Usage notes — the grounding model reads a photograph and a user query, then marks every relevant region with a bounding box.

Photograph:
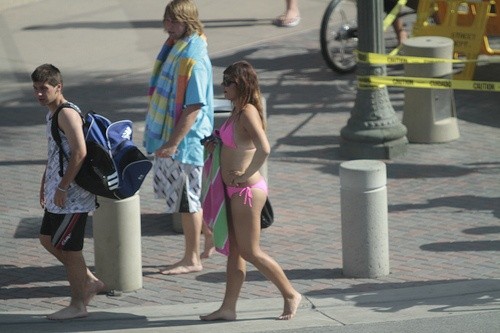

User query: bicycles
[319,0,359,75]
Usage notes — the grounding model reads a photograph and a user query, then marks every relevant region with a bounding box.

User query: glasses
[221,80,235,86]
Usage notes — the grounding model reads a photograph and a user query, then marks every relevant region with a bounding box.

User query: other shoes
[278,14,300,26]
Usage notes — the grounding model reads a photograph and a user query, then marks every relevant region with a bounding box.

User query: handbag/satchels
[261,199,273,228]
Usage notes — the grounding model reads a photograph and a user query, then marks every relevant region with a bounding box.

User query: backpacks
[49,102,152,209]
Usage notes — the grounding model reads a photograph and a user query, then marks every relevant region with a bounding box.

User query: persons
[30,63,105,322]
[197,59,304,321]
[271,0,302,27]
[380,1,422,45]
[142,0,218,275]
